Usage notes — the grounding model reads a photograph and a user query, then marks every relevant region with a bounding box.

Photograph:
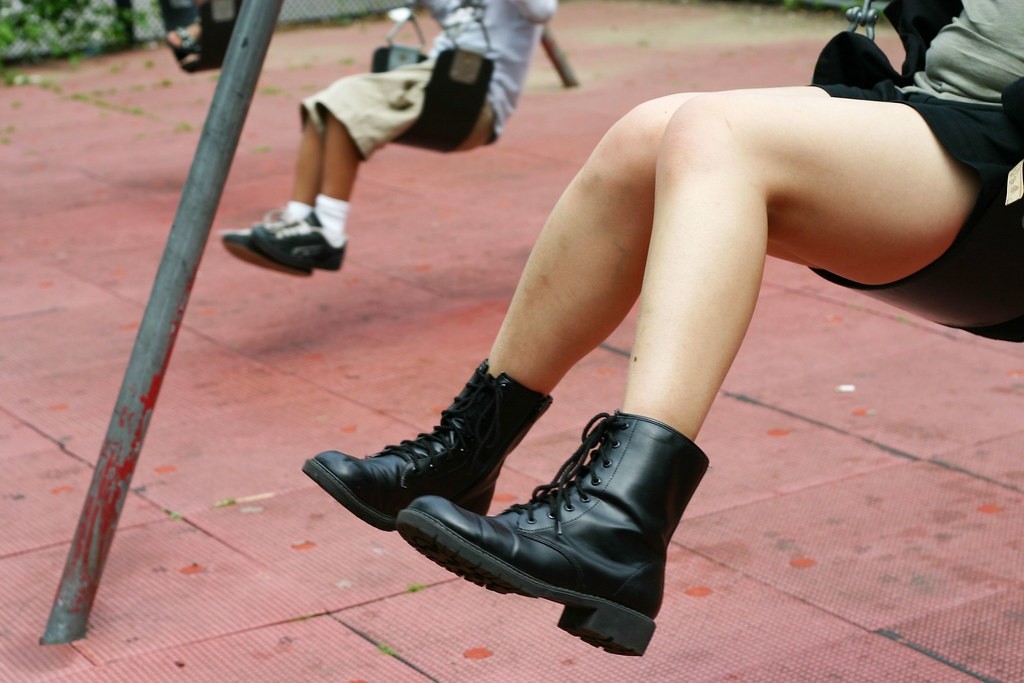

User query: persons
[302,0,1024,658]
[221,0,559,277]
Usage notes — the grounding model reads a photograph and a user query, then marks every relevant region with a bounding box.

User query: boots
[396,408,710,657]
[301,358,553,534]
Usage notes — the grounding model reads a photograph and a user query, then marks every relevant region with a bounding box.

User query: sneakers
[251,210,347,271]
[221,210,313,276]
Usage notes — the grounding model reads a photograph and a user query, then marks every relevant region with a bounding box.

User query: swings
[804,0,1024,330]
[371,1,498,155]
